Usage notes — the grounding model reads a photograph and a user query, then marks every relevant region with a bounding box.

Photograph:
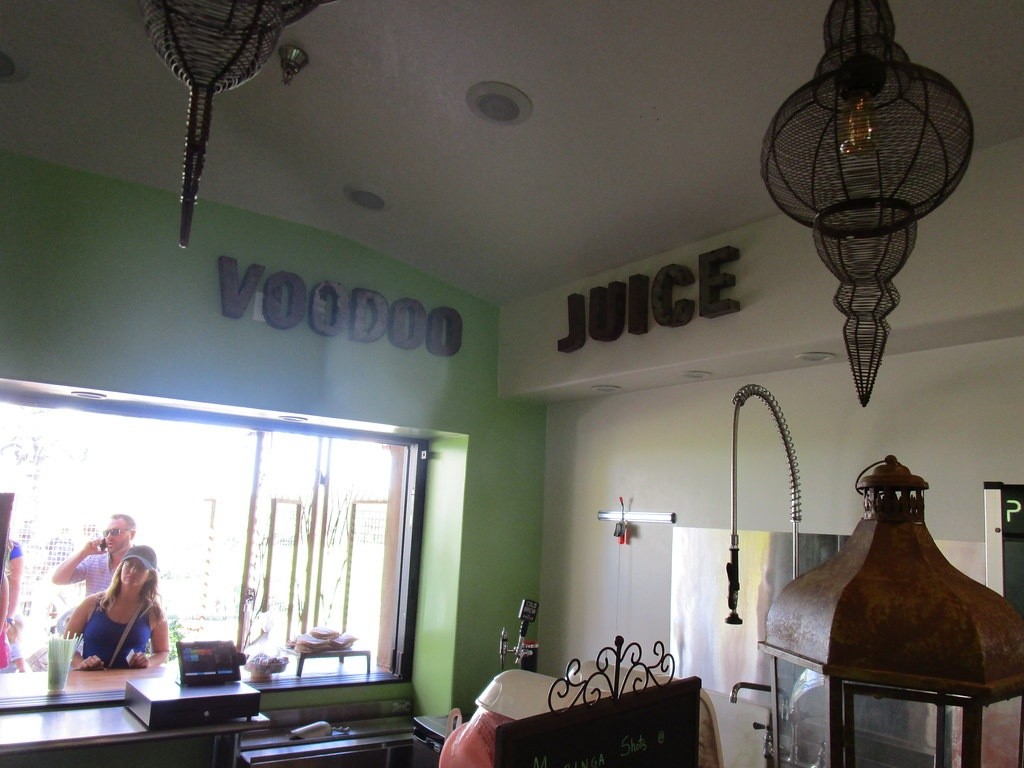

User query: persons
[0,578,10,674]
[5,540,23,631]
[0,615,25,673]
[51,513,135,596]
[62,542,170,671]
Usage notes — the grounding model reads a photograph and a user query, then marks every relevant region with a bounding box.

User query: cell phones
[100,539,107,552]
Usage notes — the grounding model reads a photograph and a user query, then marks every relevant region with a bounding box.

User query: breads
[287,627,357,654]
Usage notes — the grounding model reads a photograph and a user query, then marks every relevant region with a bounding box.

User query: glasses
[103,528,130,536]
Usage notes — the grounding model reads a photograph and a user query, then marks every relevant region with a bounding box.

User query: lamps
[598,510,676,524]
[758,0,975,408]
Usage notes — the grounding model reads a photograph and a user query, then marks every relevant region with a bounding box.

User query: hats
[121,545,158,569]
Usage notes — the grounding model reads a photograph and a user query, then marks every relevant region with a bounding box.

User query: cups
[47,654,72,695]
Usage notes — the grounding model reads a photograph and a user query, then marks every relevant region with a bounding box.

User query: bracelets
[6,618,15,625]
